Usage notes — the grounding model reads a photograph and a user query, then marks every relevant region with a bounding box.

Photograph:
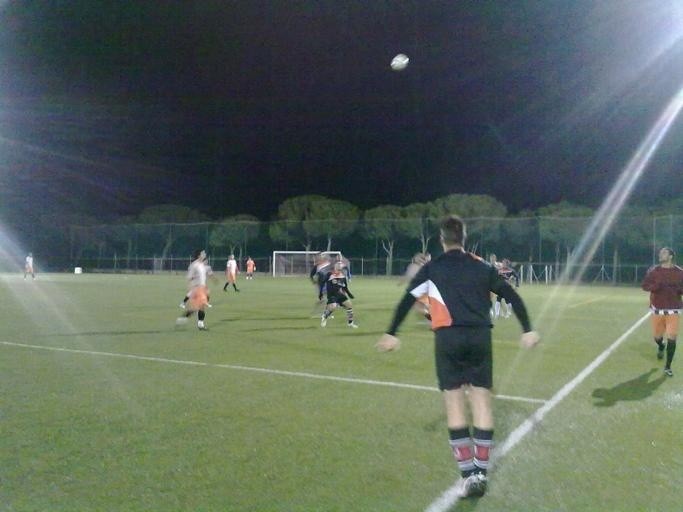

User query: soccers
[390,53,410,71]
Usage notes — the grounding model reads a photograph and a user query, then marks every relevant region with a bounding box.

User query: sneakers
[488,311,512,320]
[175,276,252,332]
[656,342,665,359]
[23,278,37,281]
[459,472,488,499]
[347,322,359,329]
[320,310,335,328]
[663,367,674,378]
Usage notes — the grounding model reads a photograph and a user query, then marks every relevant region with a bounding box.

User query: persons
[175,247,213,332]
[372,213,539,499]
[22,252,36,281]
[309,253,335,304]
[319,260,360,330]
[640,249,682,377]
[222,254,241,293]
[404,249,519,324]
[202,258,214,305]
[333,252,351,284]
[245,254,256,281]
